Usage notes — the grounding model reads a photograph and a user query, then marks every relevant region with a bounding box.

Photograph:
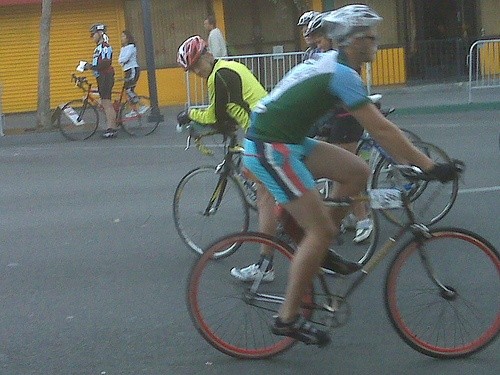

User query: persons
[241,4,456,345]
[77,23,118,138]
[118,30,151,118]
[296,11,375,242]
[203,15,229,61]
[176,34,277,282]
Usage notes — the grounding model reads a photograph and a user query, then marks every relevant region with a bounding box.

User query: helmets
[304,11,333,38]
[297,9,320,26]
[176,35,209,72]
[89,23,107,32]
[321,4,384,49]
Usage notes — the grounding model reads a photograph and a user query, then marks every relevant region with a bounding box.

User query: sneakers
[352,217,375,243]
[318,248,364,277]
[271,313,331,348]
[230,262,275,283]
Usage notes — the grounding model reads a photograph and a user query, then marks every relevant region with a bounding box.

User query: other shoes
[102,128,118,138]
[137,105,149,114]
[125,109,137,118]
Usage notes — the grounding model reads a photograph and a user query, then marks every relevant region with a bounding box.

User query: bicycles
[57,73,159,139]
[173,115,378,268]
[349,92,432,204]
[187,163,500,357]
[354,107,460,226]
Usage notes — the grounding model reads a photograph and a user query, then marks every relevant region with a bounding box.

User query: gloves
[177,110,191,126]
[431,162,462,183]
